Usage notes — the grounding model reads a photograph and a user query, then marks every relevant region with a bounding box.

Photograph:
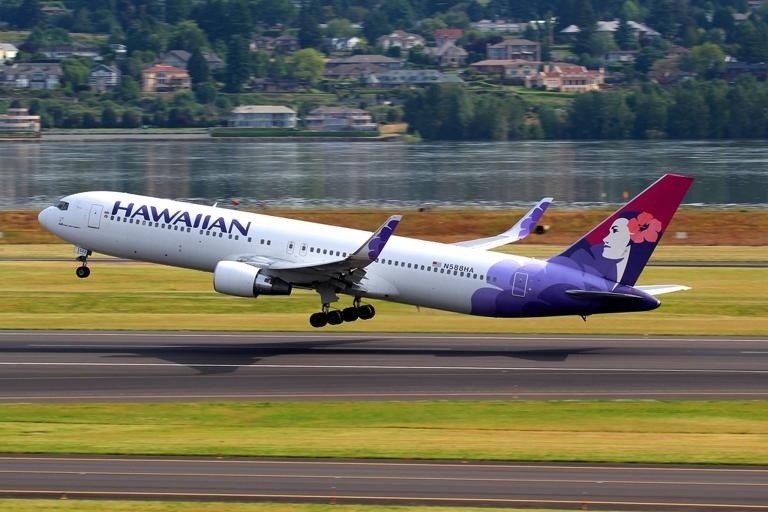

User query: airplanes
[36,172,697,330]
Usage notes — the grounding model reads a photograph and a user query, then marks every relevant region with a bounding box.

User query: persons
[601,210,663,293]
